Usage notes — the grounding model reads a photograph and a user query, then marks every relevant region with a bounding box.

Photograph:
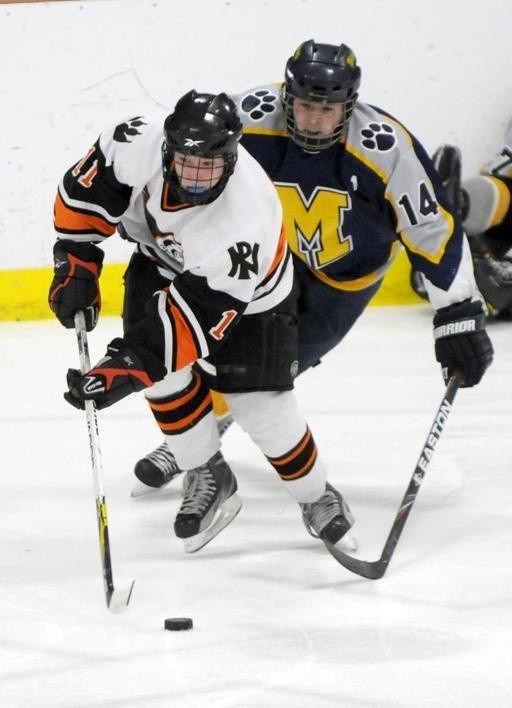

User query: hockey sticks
[322,369,461,579]
[74,310,133,613]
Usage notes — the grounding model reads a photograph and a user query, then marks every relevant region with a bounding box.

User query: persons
[45,87,356,549]
[408,137,512,319]
[132,37,494,489]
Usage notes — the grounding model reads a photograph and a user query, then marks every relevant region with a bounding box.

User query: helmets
[161,88,243,204]
[279,39,361,151]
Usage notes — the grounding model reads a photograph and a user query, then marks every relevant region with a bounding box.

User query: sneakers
[136,442,181,488]
[431,143,466,223]
[298,481,355,545]
[175,449,238,538]
[467,232,511,312]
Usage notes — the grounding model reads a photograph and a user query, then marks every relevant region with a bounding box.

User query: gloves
[49,240,105,332]
[433,300,493,388]
[64,339,167,410]
